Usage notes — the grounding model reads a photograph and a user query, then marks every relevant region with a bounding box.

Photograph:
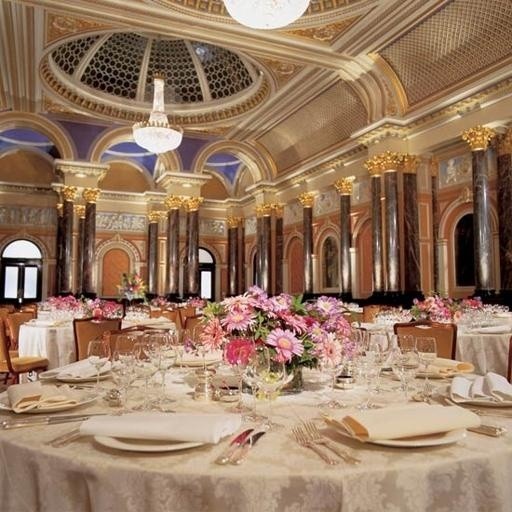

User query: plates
[92,435,208,455]
[321,423,468,448]
[401,357,511,409]
[57,373,110,383]
[1,383,105,411]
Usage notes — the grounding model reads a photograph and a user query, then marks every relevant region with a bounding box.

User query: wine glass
[86,328,437,429]
[36,297,150,325]
[375,294,511,325]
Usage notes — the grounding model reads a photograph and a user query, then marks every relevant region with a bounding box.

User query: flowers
[195,283,359,373]
[114,268,150,297]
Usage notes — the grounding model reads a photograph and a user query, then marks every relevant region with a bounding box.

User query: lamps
[221,0,311,31]
[128,30,186,157]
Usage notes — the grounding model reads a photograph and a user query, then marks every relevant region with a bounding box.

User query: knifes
[232,429,265,465]
[0,411,108,430]
[215,427,255,463]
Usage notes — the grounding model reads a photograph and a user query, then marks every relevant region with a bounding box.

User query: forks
[302,420,359,464]
[289,425,337,464]
